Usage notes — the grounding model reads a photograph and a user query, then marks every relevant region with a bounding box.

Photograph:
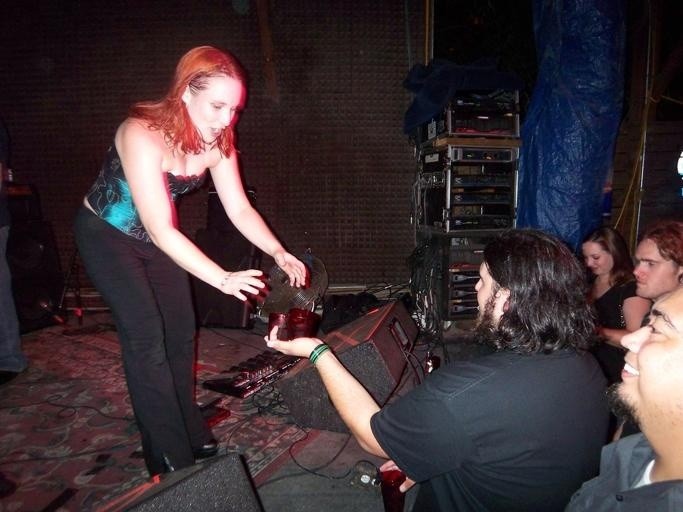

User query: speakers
[276,300,418,434]
[103,450,261,512]
[3,221,58,335]
[195,229,259,329]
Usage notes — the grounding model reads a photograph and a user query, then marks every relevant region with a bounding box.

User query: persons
[564,282,683,512]
[74,45,307,474]
[581,225,653,386]
[0,122,30,389]
[264,229,611,512]
[611,219,683,446]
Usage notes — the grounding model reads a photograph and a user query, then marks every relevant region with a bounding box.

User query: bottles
[423,350,434,377]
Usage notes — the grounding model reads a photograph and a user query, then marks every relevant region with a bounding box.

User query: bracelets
[309,343,331,364]
[218,270,236,293]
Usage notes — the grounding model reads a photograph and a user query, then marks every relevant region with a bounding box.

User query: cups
[377,470,407,511]
[266,309,321,346]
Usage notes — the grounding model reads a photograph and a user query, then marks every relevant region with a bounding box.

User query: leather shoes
[193,439,217,457]
